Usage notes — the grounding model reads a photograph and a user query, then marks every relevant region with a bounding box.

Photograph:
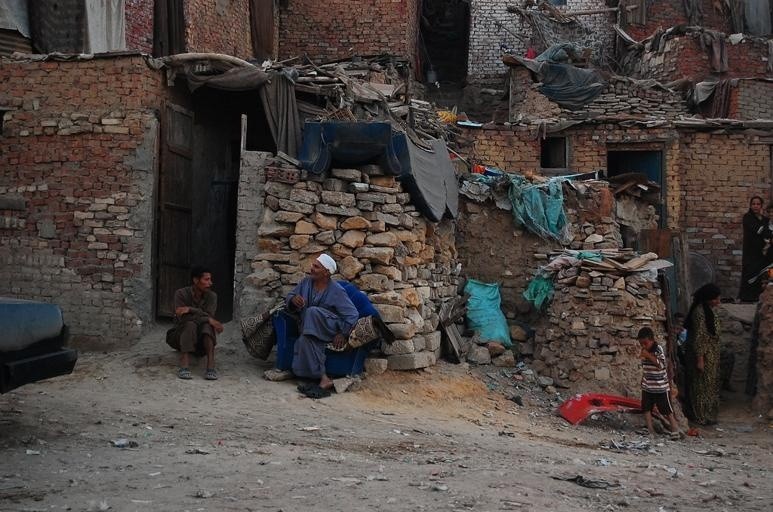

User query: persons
[673,312,686,362]
[637,327,681,440]
[737,196,770,301]
[264,253,359,390]
[165,266,222,380]
[766,203,772,264]
[683,284,720,425]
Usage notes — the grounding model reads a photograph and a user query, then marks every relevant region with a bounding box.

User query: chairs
[270,279,382,378]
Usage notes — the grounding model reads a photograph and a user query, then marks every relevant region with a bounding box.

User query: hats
[316,253,337,275]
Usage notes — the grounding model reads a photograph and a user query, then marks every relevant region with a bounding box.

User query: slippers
[204,367,218,380]
[177,367,193,380]
[297,382,331,399]
